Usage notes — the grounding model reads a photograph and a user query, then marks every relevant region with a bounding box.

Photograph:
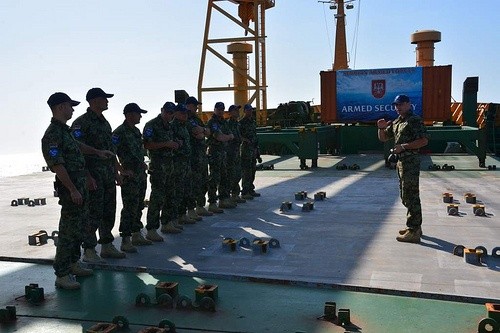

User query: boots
[161,221,181,233]
[396,225,421,242]
[83,248,105,264]
[399,225,422,236]
[120,236,137,253]
[55,274,80,289]
[132,232,152,246]
[70,260,93,277]
[146,229,163,242]
[177,190,261,224]
[100,242,127,258]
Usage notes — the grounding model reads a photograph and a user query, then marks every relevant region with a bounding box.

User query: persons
[144,103,181,241]
[377,96,429,242]
[71,87,127,262]
[41,93,99,291]
[176,95,261,226]
[111,102,152,252]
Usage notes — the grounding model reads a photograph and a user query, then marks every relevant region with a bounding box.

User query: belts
[398,151,416,159]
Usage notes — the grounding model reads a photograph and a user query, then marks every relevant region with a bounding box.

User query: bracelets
[379,128,386,130]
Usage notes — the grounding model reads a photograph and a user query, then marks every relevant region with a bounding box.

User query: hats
[186,98,202,105]
[123,103,147,114]
[164,102,175,113]
[86,88,114,101]
[391,94,410,105]
[47,93,80,107]
[215,102,225,109]
[244,104,252,109]
[175,104,191,112]
[229,105,241,111]
